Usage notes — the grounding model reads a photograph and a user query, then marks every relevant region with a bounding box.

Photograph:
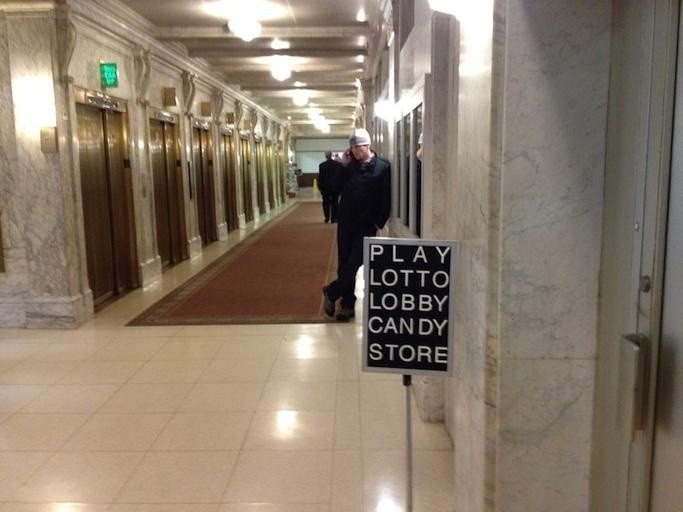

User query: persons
[317,150,343,224]
[322,127,391,323]
[333,154,341,162]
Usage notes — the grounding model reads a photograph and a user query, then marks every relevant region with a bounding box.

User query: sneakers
[336,303,355,321]
[322,283,336,317]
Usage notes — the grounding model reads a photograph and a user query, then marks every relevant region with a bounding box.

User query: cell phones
[347,151,354,158]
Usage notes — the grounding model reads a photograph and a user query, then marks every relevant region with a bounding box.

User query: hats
[348,127,372,149]
